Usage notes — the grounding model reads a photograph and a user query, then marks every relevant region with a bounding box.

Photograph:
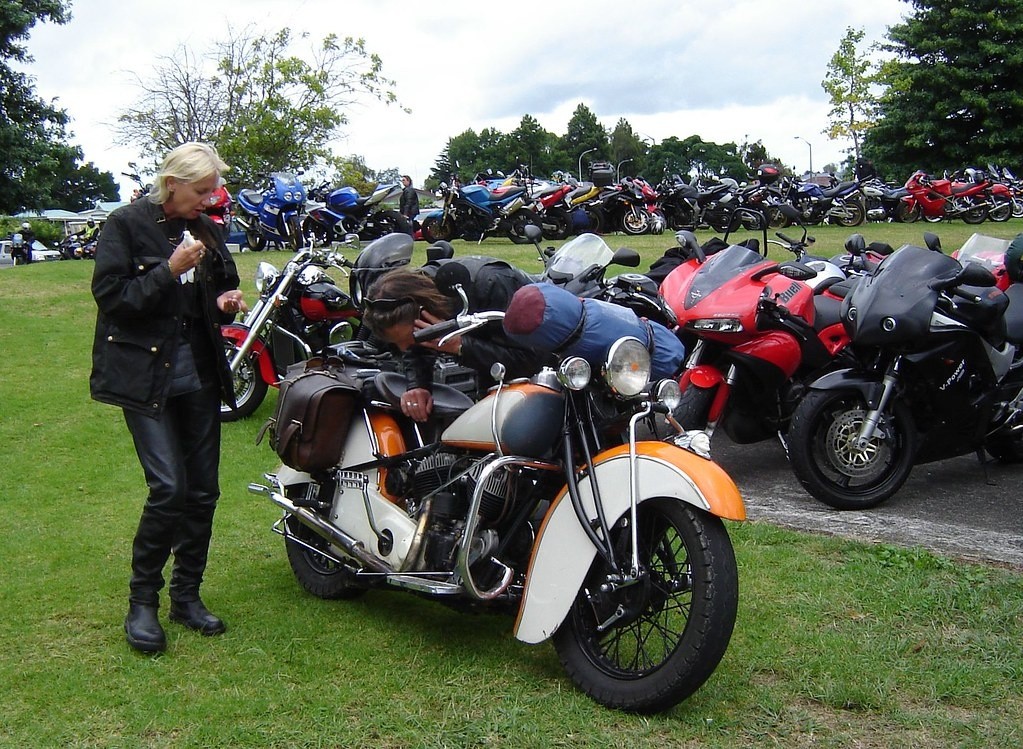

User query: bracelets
[459,345,462,355]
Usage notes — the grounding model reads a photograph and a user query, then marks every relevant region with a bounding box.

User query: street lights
[579,147,598,187]
[617,158,634,184]
[794,136,813,183]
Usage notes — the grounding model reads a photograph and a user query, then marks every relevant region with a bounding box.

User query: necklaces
[169,218,187,240]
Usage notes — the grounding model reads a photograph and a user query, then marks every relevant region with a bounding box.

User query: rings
[407,402,412,406]
[413,403,417,406]
[199,250,202,259]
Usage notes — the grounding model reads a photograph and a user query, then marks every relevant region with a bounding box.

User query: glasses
[363,295,411,314]
[303,356,348,374]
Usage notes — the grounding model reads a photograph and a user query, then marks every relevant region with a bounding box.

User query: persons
[400,176,420,240]
[16,222,35,264]
[856,157,876,179]
[70,218,99,246]
[362,256,558,423]
[207,177,232,235]
[90,141,248,650]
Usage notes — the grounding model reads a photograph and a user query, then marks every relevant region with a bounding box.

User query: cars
[0,238,63,265]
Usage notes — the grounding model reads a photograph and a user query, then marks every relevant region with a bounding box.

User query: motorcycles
[53,159,154,262]
[780,232,1023,511]
[6,231,31,266]
[198,159,1023,422]
[246,262,749,718]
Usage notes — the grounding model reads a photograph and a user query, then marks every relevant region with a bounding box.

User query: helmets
[22,222,32,233]
[973,170,985,184]
[87,218,94,228]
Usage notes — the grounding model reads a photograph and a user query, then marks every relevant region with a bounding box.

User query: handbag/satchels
[268,370,365,472]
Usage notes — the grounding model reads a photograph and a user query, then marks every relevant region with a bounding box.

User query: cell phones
[417,306,434,330]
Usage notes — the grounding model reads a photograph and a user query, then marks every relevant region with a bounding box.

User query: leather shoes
[123,602,167,650]
[168,597,223,635]
